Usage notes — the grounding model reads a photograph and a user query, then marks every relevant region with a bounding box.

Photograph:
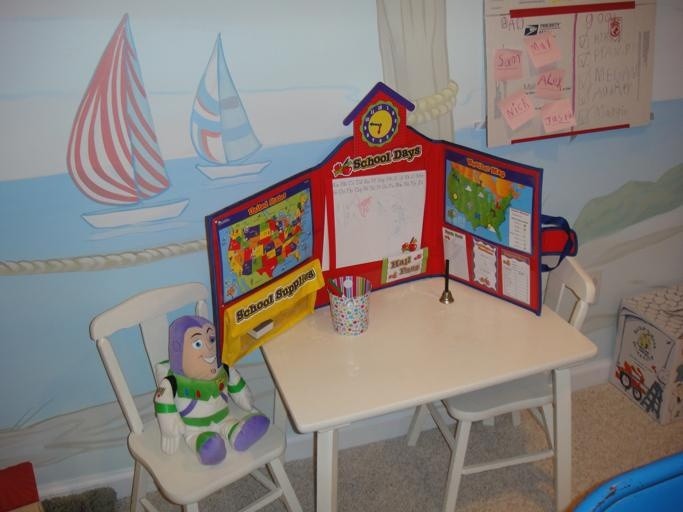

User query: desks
[260,276,599,511]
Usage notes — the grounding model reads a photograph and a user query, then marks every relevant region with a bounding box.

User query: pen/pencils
[326,278,353,298]
[445,260,449,292]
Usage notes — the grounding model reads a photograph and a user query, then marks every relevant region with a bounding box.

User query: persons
[154,315,270,464]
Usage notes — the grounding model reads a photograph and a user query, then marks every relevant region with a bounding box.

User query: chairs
[409,252,595,511]
[91,283,303,512]
